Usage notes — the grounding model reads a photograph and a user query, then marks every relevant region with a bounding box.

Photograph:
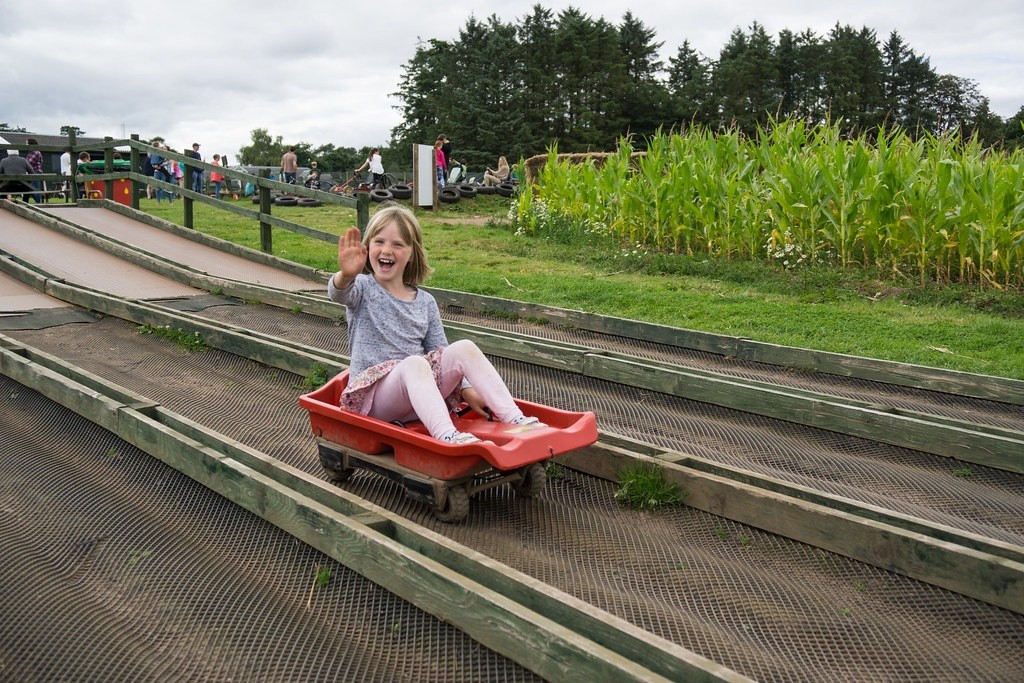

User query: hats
[193,143,201,146]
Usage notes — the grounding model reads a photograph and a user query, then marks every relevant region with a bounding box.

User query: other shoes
[520,417,548,427]
[454,433,495,445]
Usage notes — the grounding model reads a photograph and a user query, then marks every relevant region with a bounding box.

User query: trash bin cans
[79,159,133,205]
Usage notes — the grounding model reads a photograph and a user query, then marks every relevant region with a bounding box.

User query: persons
[355,148,385,185]
[510,163,519,180]
[280,146,298,194]
[304,161,321,189]
[434,140,448,190]
[114,153,122,159]
[190,143,204,194]
[24,139,44,203]
[74,151,91,200]
[142,142,185,205]
[481,156,510,187]
[0,149,35,204]
[209,154,223,199]
[436,133,450,170]
[328,202,548,446]
[58,151,72,199]
